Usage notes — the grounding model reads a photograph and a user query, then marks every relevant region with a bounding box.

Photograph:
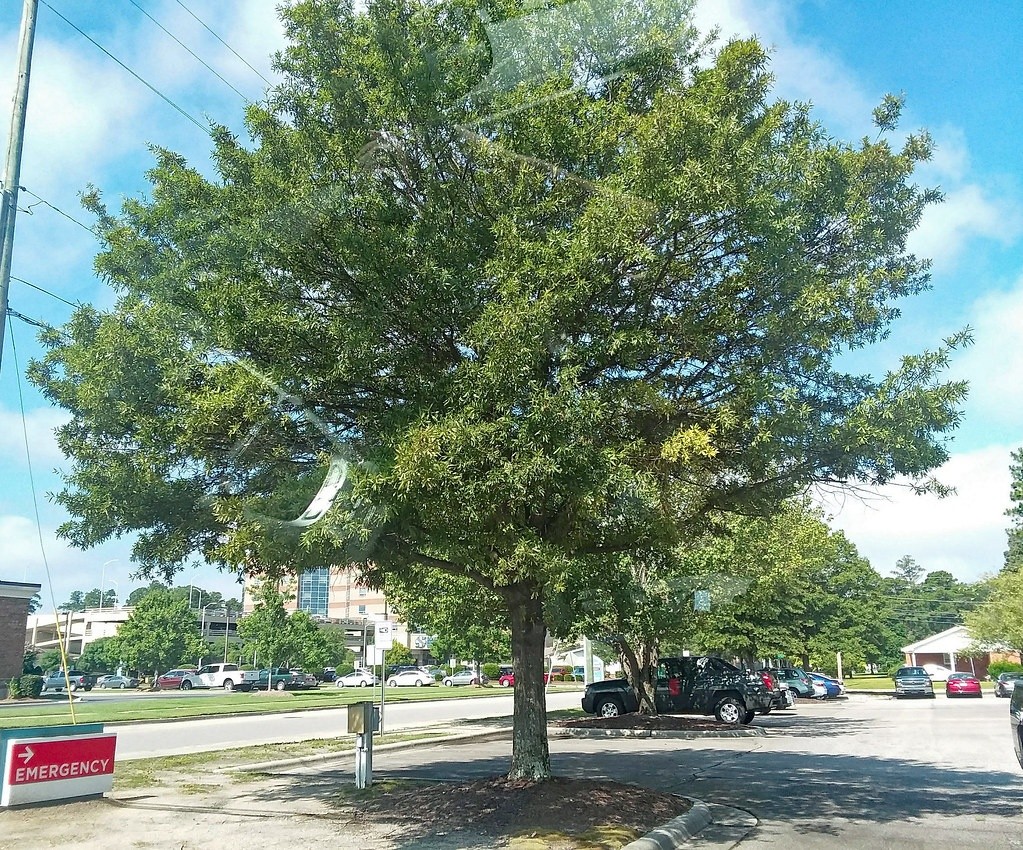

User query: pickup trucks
[181,662,259,692]
[250,667,306,690]
[41,669,95,692]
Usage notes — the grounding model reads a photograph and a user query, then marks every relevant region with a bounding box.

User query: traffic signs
[0,732,118,808]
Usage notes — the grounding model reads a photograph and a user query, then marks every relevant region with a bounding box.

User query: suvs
[891,666,935,699]
[581,656,783,726]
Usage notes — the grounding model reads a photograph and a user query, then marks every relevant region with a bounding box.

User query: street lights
[198,603,217,670]
[363,622,375,668]
[99,558,120,613]
[108,580,118,611]
[61,611,69,669]
[193,587,201,612]
[189,574,200,609]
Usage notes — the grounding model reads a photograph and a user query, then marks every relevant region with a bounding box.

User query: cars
[99,675,137,689]
[943,672,982,698]
[149,668,198,690]
[994,672,1023,698]
[97,674,115,686]
[298,673,317,691]
[754,666,846,716]
[322,665,585,688]
